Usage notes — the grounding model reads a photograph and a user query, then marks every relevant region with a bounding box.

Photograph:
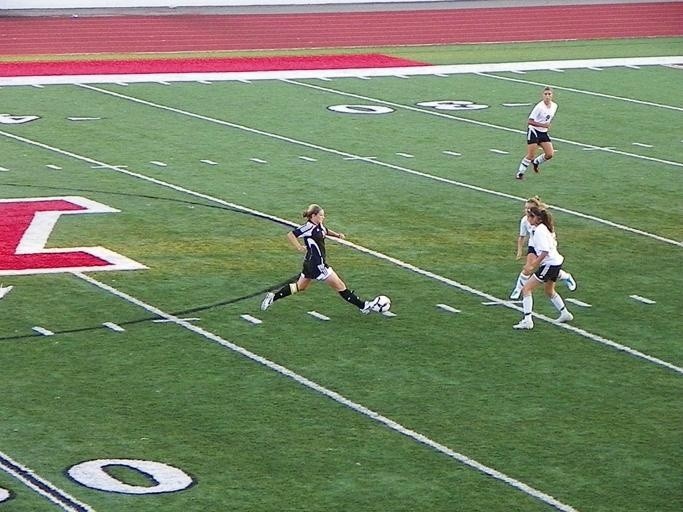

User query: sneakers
[556,313,573,323]
[516,172,523,179]
[260,292,275,311]
[360,296,380,314]
[510,287,522,300]
[567,272,577,291]
[512,319,534,329]
[531,160,538,173]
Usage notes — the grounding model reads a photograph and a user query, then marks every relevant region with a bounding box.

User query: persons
[516,87,558,179]
[513,207,573,329]
[510,196,576,299]
[261,204,380,315]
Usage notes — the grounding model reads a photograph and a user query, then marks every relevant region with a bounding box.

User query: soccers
[372,296,391,312]
